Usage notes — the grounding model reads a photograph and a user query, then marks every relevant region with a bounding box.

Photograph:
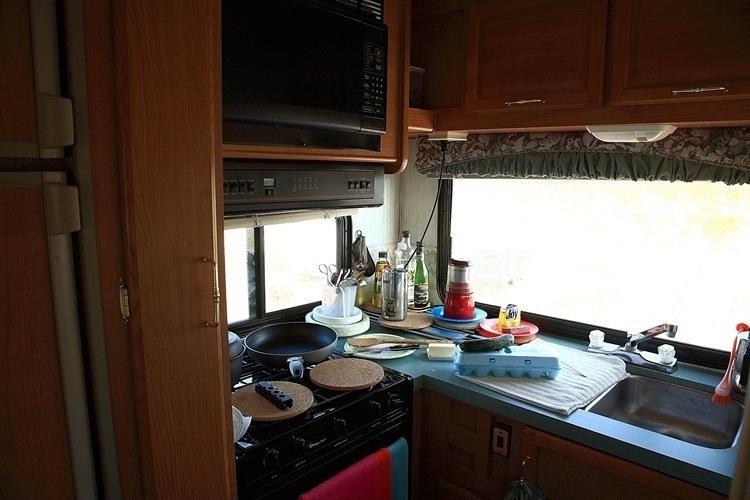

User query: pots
[228,322,338,386]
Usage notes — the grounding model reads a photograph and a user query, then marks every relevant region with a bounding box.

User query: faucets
[624,322,678,351]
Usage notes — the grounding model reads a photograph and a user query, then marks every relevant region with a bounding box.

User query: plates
[345,333,416,359]
[431,306,539,337]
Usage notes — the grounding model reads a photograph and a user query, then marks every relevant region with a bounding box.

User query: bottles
[372,230,428,308]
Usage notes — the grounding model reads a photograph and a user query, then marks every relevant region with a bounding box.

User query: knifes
[343,344,421,355]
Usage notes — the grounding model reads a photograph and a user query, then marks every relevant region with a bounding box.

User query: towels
[454,337,633,418]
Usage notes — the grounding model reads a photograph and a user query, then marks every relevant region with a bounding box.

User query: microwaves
[222,1,388,137]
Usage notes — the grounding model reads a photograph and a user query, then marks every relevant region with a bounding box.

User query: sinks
[585,374,746,449]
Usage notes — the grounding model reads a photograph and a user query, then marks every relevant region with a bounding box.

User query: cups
[322,286,357,318]
[381,268,408,321]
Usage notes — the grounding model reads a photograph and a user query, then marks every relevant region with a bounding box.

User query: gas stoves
[231,339,406,483]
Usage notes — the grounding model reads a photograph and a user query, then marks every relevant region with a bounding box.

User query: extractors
[223,158,385,215]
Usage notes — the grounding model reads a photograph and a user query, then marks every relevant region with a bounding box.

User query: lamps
[584,124,679,144]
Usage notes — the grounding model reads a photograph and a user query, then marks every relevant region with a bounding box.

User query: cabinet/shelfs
[424,393,491,499]
[461,0,750,116]
[517,427,728,500]
